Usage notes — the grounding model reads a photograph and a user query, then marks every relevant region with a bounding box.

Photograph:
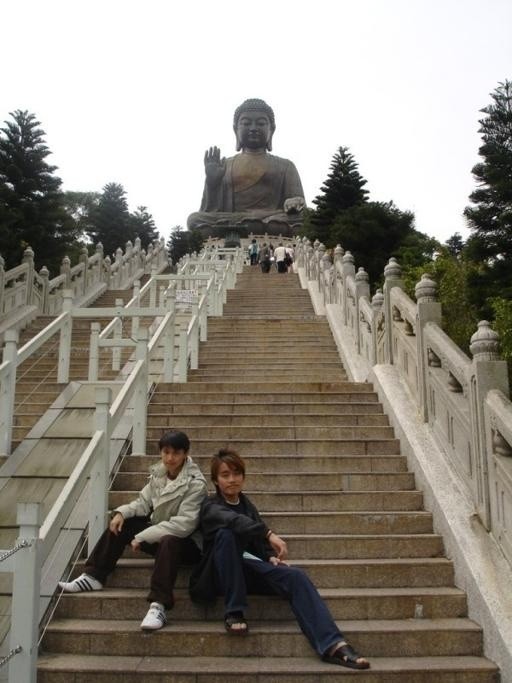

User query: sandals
[322,644,369,669]
[224,610,248,633]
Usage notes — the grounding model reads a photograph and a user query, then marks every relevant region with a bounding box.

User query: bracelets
[267,531,273,540]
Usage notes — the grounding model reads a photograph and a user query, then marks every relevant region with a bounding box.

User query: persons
[187,99,314,239]
[248,239,296,273]
[188,449,370,669]
[57,430,208,631]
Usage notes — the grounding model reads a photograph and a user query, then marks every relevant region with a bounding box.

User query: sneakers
[140,602,167,629]
[58,573,103,593]
